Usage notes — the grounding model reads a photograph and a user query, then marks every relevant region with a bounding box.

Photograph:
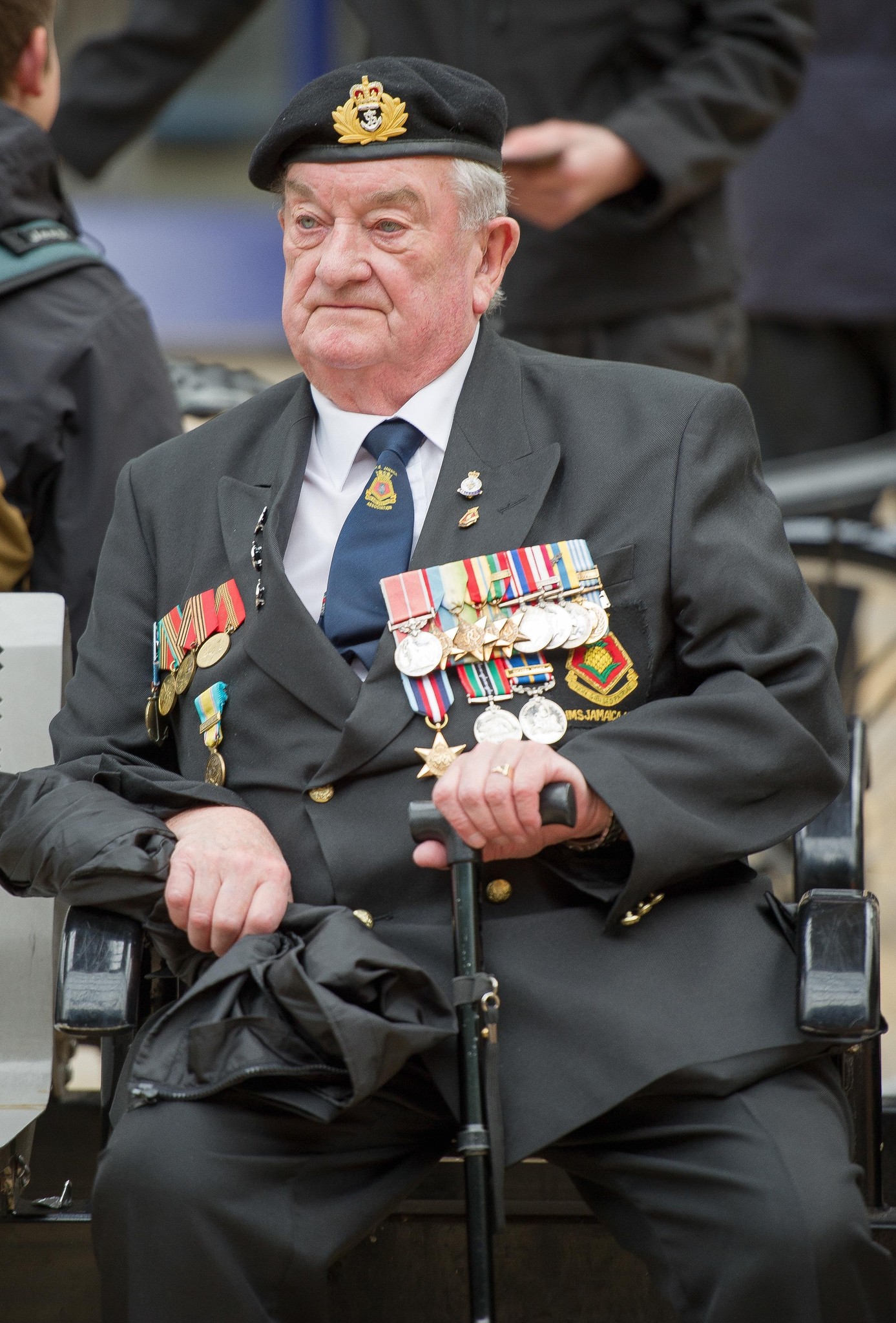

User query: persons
[0,0,816,687]
[46,54,896,1323]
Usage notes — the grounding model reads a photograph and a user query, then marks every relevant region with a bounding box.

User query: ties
[315,418,426,675]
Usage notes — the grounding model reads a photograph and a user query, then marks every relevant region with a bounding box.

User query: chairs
[52,708,885,1205]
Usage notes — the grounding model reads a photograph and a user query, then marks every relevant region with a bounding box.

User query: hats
[249,57,509,193]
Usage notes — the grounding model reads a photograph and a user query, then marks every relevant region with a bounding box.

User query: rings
[487,765,515,779]
